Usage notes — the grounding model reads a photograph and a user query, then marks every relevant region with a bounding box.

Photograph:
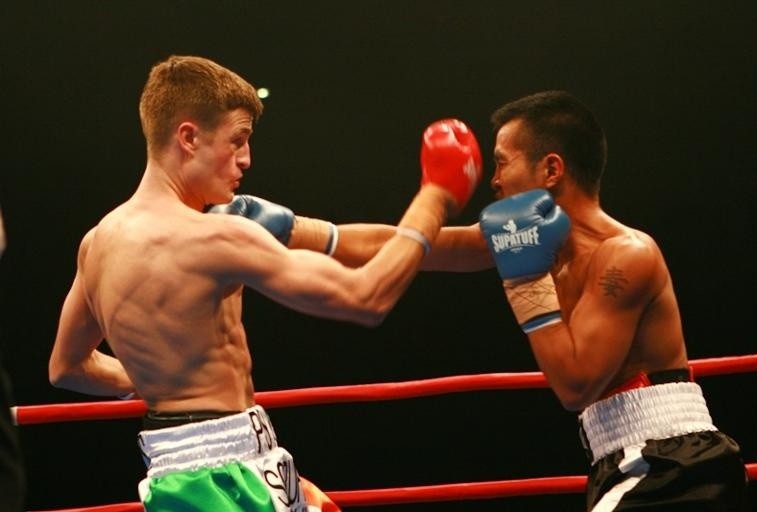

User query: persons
[209,90,756,512]
[45,51,484,511]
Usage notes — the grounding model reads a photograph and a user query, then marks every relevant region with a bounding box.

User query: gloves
[211,187,338,256]
[398,117,481,255]
[477,189,572,333]
